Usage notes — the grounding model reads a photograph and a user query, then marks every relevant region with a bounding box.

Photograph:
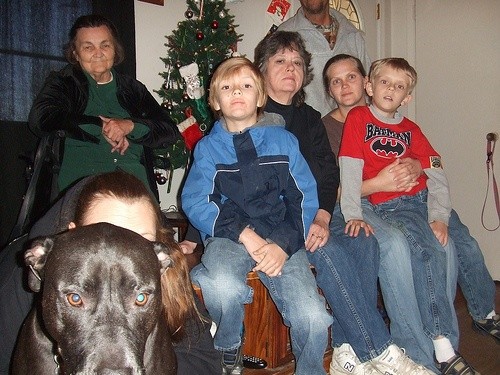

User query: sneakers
[432,349,482,375]
[473,312,499,342]
[328,343,437,375]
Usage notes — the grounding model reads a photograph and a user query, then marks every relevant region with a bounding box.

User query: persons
[180,0,498,374]
[1,169,224,375]
[5,13,181,246]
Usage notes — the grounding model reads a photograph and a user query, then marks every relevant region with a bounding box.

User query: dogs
[8,222,178,375]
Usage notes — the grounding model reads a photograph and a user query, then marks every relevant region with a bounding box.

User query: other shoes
[220,323,244,374]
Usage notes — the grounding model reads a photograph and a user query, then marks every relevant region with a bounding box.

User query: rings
[312,233,318,239]
[317,236,323,241]
